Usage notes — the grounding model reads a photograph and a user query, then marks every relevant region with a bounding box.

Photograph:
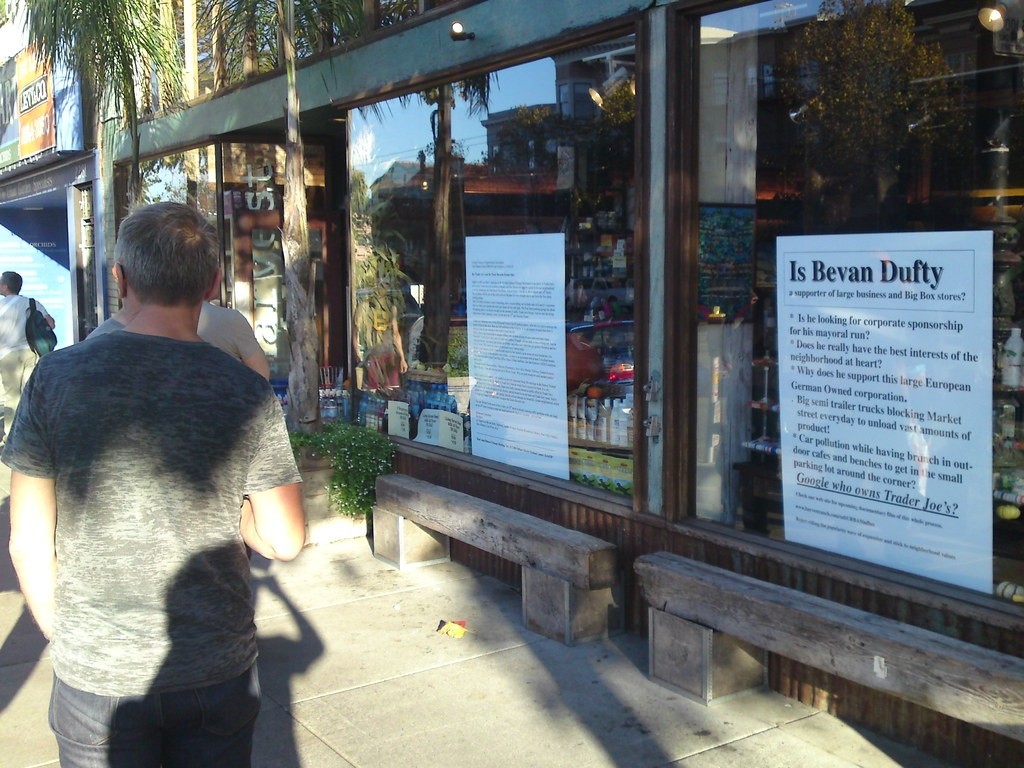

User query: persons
[85,307,127,341]
[1,272,57,444]
[0,203,308,768]
[197,300,270,381]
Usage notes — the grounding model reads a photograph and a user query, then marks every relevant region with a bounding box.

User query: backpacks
[24,297,57,357]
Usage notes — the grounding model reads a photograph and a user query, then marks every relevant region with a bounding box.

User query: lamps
[976,0,1013,33]
[789,90,826,123]
[449,21,476,41]
[907,112,939,136]
[588,67,637,112]
[987,113,1014,152]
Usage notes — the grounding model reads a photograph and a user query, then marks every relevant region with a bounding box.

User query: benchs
[373,474,625,647]
[633,550,1024,743]
